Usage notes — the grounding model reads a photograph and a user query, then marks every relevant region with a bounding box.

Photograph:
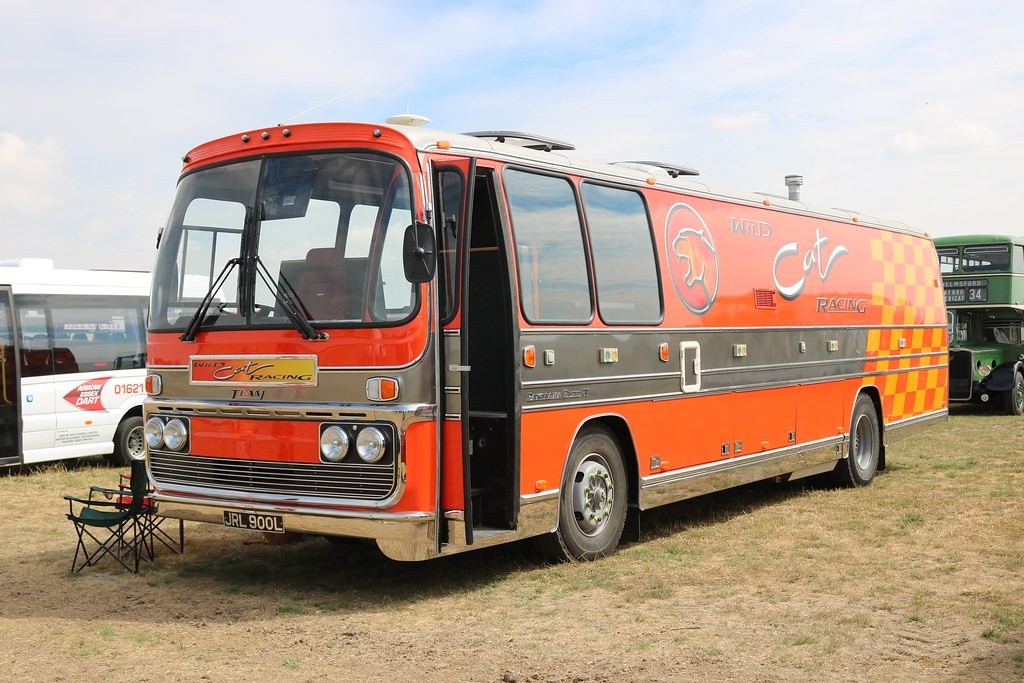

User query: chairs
[0,332,126,405]
[295,245,347,320]
[66,457,187,574]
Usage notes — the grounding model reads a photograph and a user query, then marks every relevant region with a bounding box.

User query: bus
[142,114,951,564]
[932,235,1024,415]
[0,256,227,471]
